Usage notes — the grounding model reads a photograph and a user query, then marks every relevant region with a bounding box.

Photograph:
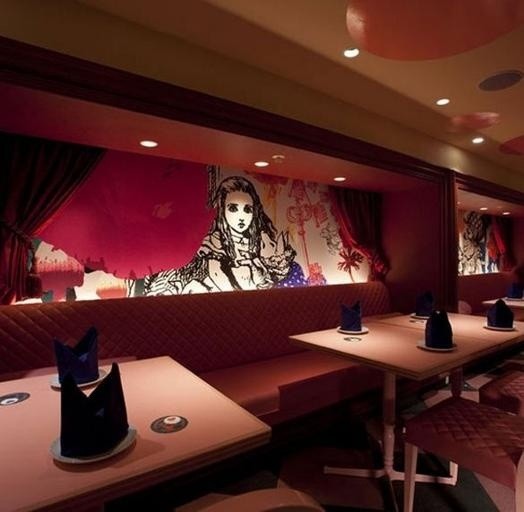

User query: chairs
[398,389,521,511]
[477,353,524,413]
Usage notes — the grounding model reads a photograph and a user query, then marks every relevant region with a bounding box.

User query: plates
[483,324,514,330]
[417,340,458,352]
[336,325,369,335]
[410,312,431,318]
[48,427,138,464]
[50,369,110,389]
[505,297,522,300]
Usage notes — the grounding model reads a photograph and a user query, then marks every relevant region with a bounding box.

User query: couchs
[0,280,395,429]
[457,271,515,313]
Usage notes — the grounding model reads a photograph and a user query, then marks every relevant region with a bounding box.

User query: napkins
[486,297,515,330]
[412,289,432,317]
[423,311,454,349]
[339,301,362,330]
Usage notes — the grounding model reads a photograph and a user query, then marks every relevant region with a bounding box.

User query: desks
[0,353,271,512]
[288,321,496,485]
[383,312,523,391]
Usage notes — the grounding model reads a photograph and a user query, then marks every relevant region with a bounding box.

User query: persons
[143,176,296,296]
